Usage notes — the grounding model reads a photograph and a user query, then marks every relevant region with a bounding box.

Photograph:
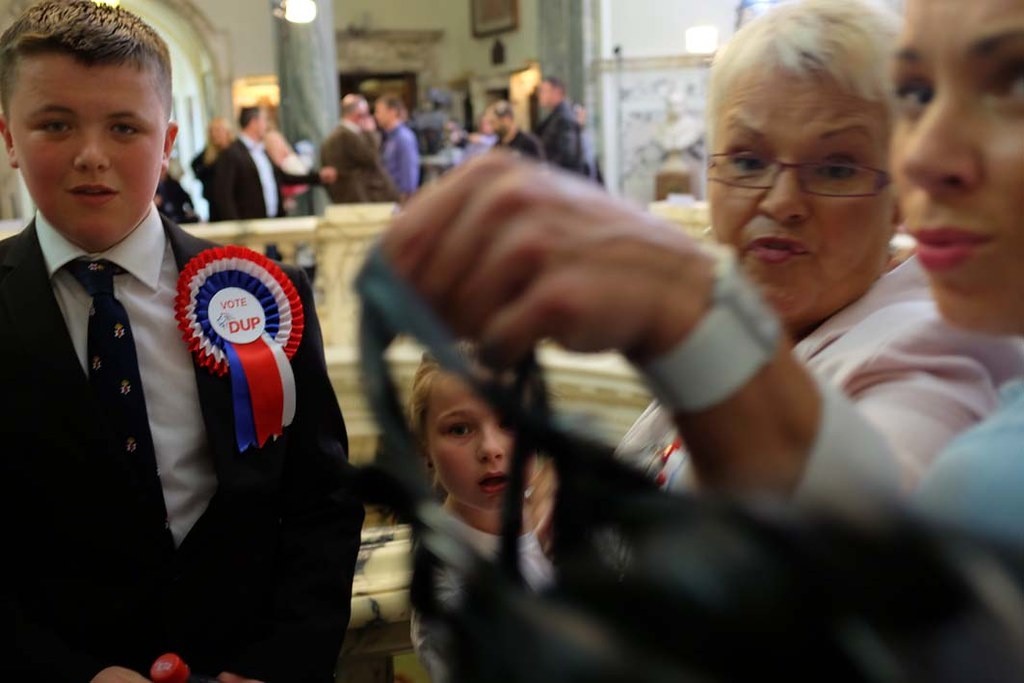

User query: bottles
[151,654,203,683]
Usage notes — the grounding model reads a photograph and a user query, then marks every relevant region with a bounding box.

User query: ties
[63,258,175,567]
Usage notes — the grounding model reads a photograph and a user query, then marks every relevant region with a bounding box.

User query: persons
[156,74,604,227]
[0,0,366,683]
[649,81,706,152]
[409,0,1024,683]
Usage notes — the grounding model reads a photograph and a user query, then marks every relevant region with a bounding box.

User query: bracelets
[635,246,782,416]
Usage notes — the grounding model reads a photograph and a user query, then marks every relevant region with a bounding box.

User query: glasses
[707,152,890,197]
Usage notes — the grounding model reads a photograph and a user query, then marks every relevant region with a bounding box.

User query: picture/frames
[471,1,517,40]
